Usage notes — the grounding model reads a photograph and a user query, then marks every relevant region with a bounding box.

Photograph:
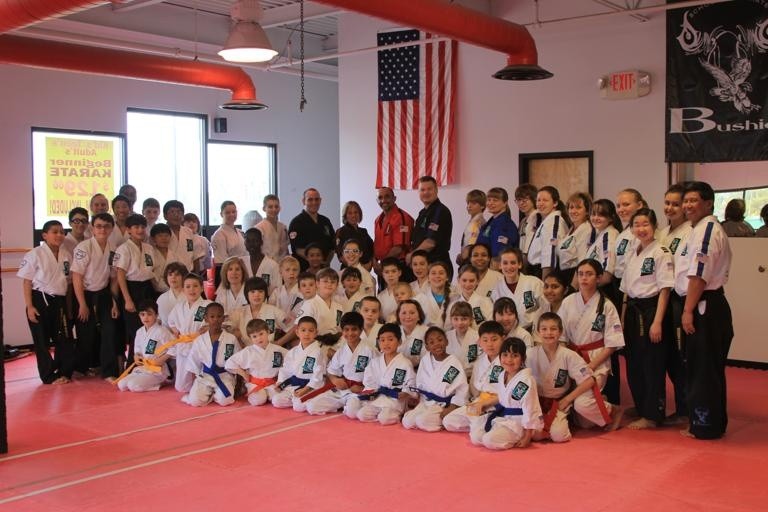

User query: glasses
[71,218,89,224]
[513,198,529,204]
[290,302,304,319]
[577,272,597,279]
[343,248,360,256]
[93,223,111,230]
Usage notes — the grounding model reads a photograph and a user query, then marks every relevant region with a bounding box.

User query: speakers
[215,118,226,132]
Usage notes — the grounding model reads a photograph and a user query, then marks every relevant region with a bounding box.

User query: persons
[16,174,214,385]
[754,203,768,237]
[611,179,735,440]
[300,175,626,449]
[116,186,336,415]
[720,198,754,238]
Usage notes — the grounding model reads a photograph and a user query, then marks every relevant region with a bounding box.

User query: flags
[373,26,460,190]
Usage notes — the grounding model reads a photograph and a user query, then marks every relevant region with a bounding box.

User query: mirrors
[711,186,768,238]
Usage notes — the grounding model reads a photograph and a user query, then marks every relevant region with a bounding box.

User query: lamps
[217,2,281,65]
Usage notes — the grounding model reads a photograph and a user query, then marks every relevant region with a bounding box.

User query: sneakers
[2,344,19,359]
